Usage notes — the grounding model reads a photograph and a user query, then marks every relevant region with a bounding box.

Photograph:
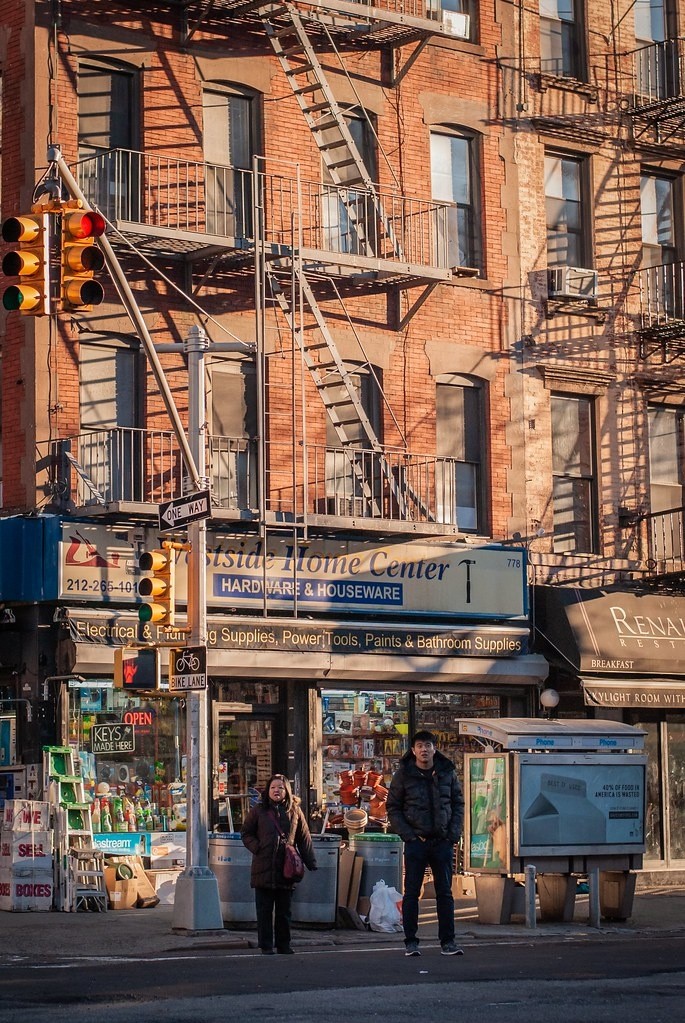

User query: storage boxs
[0,798,54,912]
[79,688,102,711]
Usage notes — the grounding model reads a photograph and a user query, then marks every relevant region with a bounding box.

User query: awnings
[532,589,685,710]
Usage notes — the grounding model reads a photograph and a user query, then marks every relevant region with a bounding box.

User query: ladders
[43,746,108,913]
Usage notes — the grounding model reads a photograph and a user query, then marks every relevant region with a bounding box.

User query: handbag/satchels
[284,844,304,882]
[369,881,403,932]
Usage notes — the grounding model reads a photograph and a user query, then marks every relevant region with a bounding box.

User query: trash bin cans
[289,833,342,930]
[473,875,516,925]
[599,871,637,920]
[209,833,259,928]
[536,873,578,921]
[349,833,403,897]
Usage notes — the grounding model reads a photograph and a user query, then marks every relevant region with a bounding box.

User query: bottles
[90,780,176,834]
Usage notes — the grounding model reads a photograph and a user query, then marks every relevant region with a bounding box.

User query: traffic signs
[157,488,213,533]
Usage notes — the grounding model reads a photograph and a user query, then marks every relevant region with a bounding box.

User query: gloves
[308,860,319,871]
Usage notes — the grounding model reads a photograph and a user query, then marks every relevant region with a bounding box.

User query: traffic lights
[0,213,50,317]
[114,647,160,691]
[138,547,175,627]
[57,205,106,312]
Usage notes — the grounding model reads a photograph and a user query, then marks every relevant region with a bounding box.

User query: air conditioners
[427,8,470,40]
[547,266,597,301]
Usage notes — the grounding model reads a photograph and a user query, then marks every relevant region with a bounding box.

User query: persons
[385,730,465,956]
[240,774,318,954]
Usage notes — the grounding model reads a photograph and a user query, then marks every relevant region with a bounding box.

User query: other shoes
[262,949,275,955]
[277,946,295,954]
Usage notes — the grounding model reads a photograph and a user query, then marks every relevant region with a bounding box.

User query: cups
[323,716,334,733]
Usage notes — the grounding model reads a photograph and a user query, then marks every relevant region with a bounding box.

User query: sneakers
[440,941,464,955]
[405,941,422,956]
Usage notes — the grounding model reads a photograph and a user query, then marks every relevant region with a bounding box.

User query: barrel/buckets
[340,769,388,805]
[344,809,368,834]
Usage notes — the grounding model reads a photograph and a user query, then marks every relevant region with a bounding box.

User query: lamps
[541,688,559,719]
